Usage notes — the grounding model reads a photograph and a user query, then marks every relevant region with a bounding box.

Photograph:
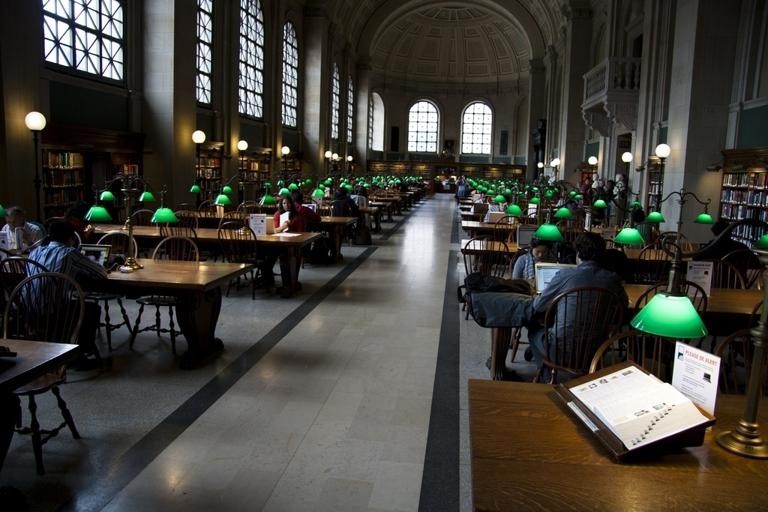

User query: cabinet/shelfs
[719,148,768,249]
[42,123,147,217]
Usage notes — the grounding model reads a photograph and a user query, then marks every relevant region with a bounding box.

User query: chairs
[0,177,436,300]
[128,236,202,353]
[3,272,85,477]
[3,256,98,364]
[95,231,139,350]
[454,177,766,391]
[38,227,82,253]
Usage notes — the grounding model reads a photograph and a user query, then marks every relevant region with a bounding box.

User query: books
[42,150,88,220]
[239,154,270,190]
[646,180,662,212]
[111,164,137,224]
[568,364,710,452]
[195,158,221,202]
[721,171,767,247]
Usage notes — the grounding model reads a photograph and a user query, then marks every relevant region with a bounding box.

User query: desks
[5,254,253,368]
[468,378,768,512]
[2,338,81,393]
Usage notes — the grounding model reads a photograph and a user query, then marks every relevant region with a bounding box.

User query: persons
[331,187,361,247]
[579,172,627,233]
[0,205,44,256]
[454,175,471,204]
[63,208,95,242]
[692,217,760,288]
[512,237,558,279]
[253,195,308,295]
[484,201,509,242]
[272,191,321,265]
[526,231,631,381]
[17,220,108,374]
[469,188,491,214]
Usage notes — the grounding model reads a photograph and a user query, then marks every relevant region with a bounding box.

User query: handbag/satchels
[352,227,372,245]
[465,272,533,329]
[309,237,329,266]
